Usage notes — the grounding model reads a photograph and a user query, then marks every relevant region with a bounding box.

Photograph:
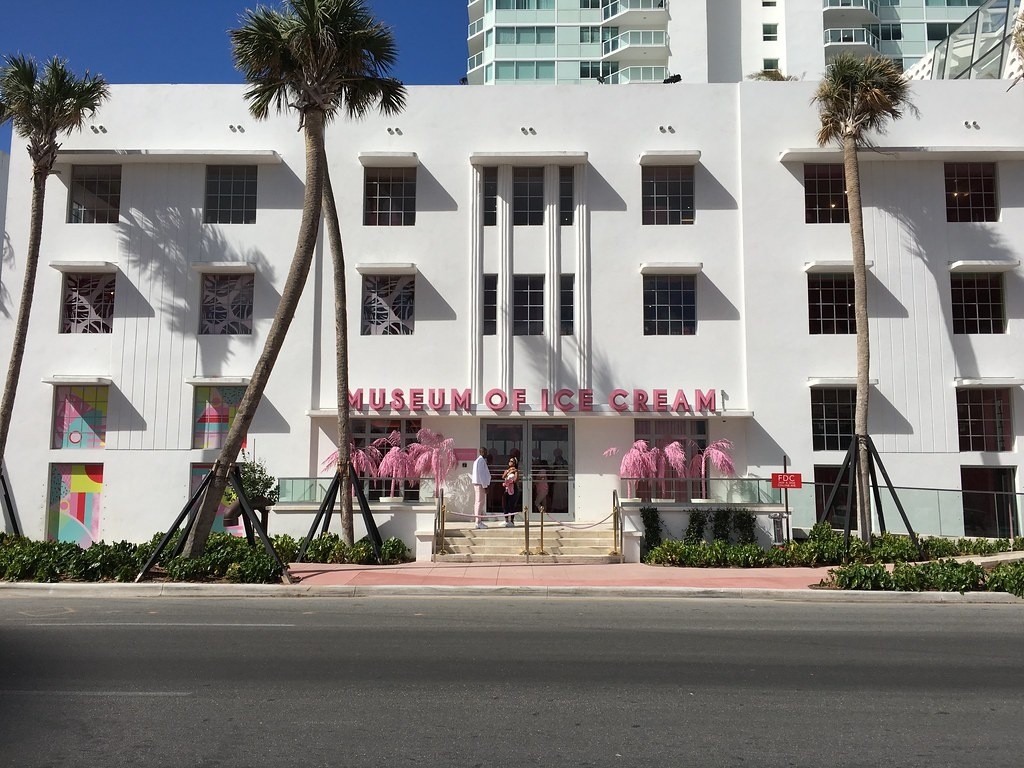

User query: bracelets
[507,470,509,473]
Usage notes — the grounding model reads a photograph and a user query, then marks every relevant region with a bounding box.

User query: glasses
[509,461,515,462]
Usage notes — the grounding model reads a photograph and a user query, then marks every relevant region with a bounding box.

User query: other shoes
[501,521,507,526]
[475,523,488,529]
[506,522,514,527]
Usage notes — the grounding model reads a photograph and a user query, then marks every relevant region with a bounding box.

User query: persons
[534,469,548,512]
[500,455,518,527]
[471,446,491,528]
[551,456,568,510]
